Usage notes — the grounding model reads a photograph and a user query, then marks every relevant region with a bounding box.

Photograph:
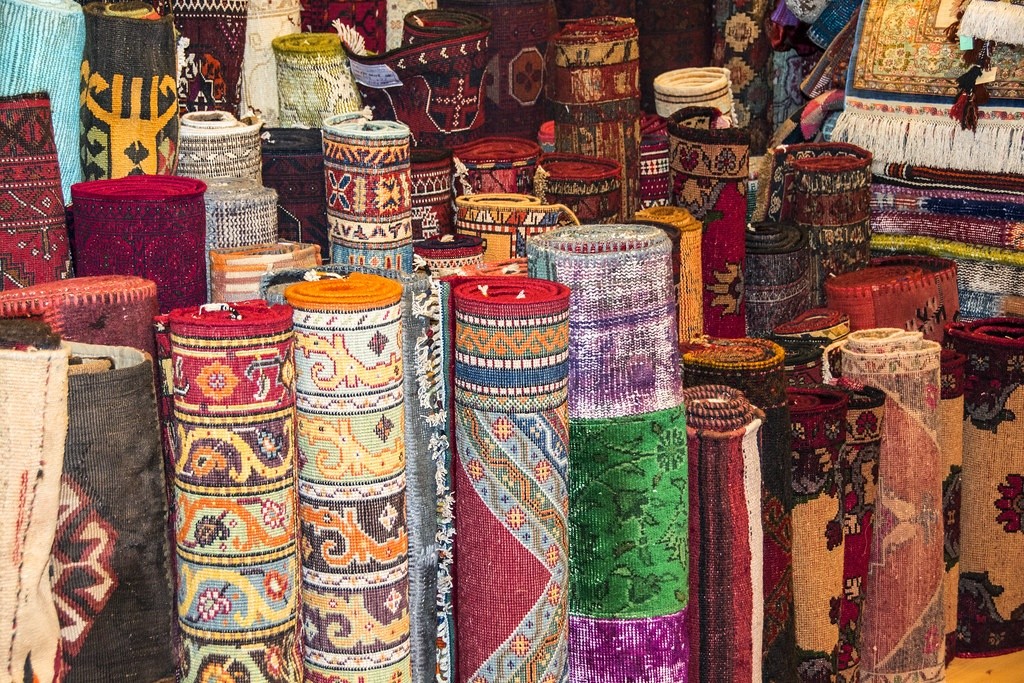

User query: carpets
[0,0,1024,683]
